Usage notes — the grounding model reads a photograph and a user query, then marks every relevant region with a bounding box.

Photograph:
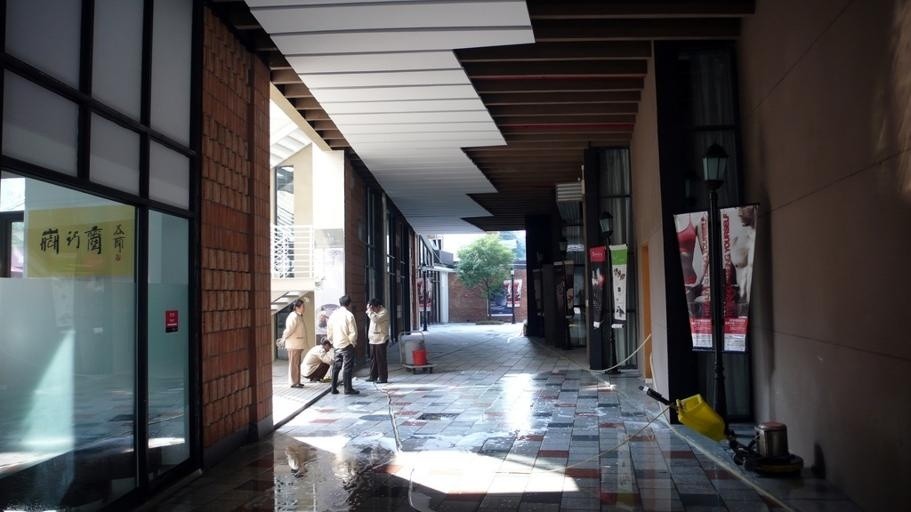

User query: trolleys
[638,384,805,478]
[398,329,436,374]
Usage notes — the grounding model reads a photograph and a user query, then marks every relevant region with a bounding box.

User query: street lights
[422,262,428,331]
[537,245,545,334]
[600,208,622,376]
[702,141,736,440]
[559,236,574,350]
[511,267,516,323]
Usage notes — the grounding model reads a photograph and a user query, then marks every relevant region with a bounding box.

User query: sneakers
[366,379,387,383]
[332,390,339,394]
[291,383,304,388]
[345,389,359,394]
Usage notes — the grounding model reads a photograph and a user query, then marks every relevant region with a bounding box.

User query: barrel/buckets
[413,350,426,366]
[404,340,423,365]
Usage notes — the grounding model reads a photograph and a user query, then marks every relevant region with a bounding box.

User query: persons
[364,298,391,383]
[673,211,709,303]
[299,338,334,383]
[286,445,311,479]
[278,300,306,389]
[327,295,361,395]
